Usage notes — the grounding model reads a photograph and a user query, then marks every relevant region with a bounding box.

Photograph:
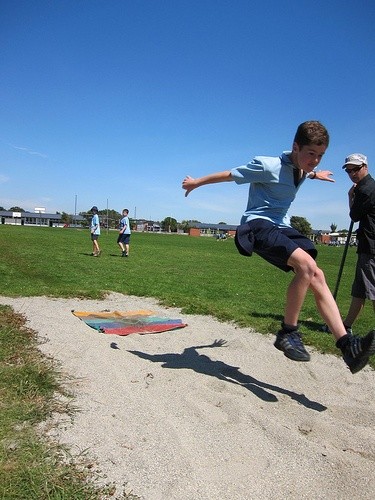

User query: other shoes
[121,250,129,257]
[91,250,101,256]
[323,323,353,335]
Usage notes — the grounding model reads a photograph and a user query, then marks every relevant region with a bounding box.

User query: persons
[117,209,131,257]
[216,233,227,241]
[89,206,101,257]
[322,154,375,334]
[182,120,375,374]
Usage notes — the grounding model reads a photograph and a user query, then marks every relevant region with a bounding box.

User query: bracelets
[310,171,316,179]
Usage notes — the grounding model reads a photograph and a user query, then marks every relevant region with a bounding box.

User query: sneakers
[273,329,310,362]
[341,330,375,374]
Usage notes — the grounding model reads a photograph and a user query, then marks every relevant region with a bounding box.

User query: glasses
[345,164,366,173]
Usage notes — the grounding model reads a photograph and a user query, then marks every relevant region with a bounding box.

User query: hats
[342,153,368,169]
